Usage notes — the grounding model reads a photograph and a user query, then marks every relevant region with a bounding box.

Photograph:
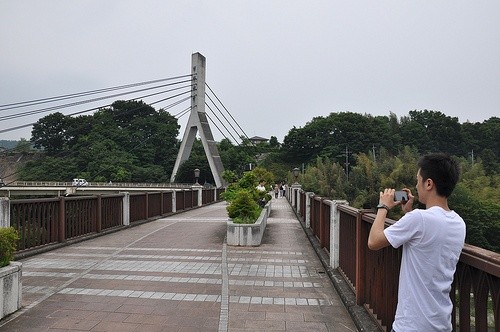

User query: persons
[368,154,467,332]
[255,179,266,209]
[270,183,286,199]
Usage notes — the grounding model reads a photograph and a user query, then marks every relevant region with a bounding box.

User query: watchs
[376,203,390,213]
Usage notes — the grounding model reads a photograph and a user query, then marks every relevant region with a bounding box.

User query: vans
[72,179,89,186]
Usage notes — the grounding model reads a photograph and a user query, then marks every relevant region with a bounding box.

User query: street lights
[293,167,301,185]
[194,168,201,186]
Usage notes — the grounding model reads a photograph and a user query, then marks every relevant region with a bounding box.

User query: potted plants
[1,226,21,320]
[221,169,267,245]
[253,168,272,216]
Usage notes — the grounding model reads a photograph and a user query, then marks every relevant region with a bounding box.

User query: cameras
[394,191,408,201]
